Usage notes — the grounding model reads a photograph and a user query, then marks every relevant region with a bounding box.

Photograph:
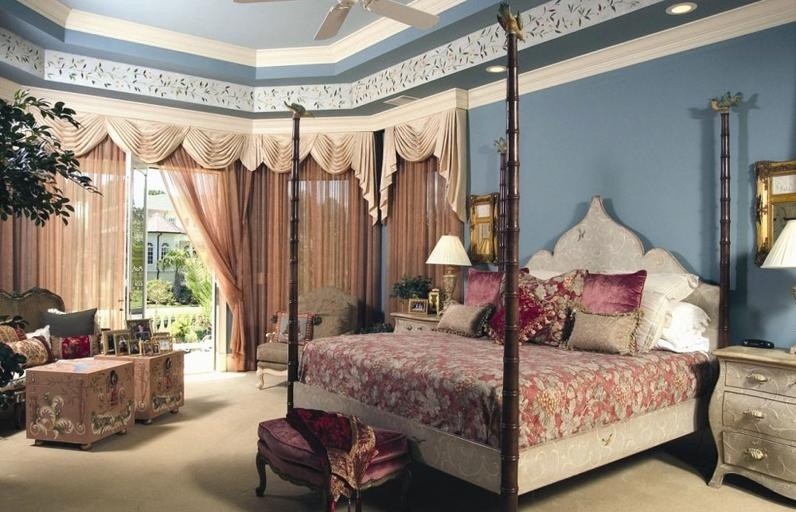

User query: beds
[281,0,747,512]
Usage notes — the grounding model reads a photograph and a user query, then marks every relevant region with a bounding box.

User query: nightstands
[388,310,445,335]
[706,337,796,506]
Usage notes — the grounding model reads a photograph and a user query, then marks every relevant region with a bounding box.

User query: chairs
[255,284,361,397]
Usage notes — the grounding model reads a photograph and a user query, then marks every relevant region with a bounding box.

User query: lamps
[421,231,473,318]
[759,216,796,358]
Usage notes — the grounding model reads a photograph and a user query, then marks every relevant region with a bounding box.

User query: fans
[232,0,441,43]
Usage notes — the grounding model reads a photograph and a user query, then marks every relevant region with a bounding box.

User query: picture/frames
[464,191,502,268]
[405,296,429,315]
[93,313,177,360]
[750,155,796,270]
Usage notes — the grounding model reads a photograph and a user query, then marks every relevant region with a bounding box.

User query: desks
[21,354,136,454]
[91,345,187,426]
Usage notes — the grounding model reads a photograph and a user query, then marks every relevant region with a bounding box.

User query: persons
[118,335,128,352]
[136,324,147,333]
[418,302,423,309]
[413,302,419,308]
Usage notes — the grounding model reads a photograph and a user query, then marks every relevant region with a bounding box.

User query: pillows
[0,303,102,373]
[297,291,354,342]
[272,307,314,348]
[430,264,717,361]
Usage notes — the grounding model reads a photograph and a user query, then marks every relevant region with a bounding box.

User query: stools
[252,411,419,511]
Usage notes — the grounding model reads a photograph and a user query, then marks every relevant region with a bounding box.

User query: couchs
[0,286,104,439]
